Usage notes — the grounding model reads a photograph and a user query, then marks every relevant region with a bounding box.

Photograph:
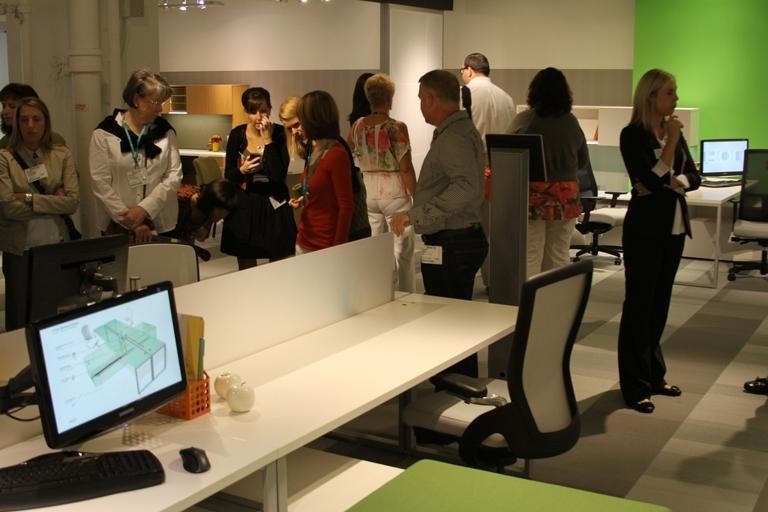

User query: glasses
[147,96,162,108]
[460,68,467,74]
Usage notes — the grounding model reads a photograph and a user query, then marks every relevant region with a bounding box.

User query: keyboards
[701,178,745,186]
[0,450,163,512]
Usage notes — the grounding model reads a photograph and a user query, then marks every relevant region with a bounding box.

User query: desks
[160,83,249,158]
[347,459,671,512]
[674,170,759,291]
[0,235,520,512]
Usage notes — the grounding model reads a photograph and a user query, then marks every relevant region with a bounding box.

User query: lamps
[0,1,31,32]
[156,1,223,11]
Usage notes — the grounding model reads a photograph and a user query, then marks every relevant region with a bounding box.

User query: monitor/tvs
[27,281,185,444]
[700,139,749,175]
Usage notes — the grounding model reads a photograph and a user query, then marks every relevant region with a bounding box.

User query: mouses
[177,445,211,474]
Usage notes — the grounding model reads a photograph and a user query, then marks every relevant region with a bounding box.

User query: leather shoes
[659,385,681,395]
[632,399,654,412]
[745,378,767,393]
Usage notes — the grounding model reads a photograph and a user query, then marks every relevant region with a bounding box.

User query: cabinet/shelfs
[515,103,699,148]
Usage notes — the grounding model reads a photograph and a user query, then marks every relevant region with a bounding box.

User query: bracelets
[25,193,33,204]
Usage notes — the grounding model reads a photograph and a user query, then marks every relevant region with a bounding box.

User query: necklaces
[248,129,266,150]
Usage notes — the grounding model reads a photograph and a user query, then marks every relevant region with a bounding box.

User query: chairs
[728,192,768,281]
[397,260,592,479]
[126,243,197,293]
[192,157,225,238]
[567,191,630,267]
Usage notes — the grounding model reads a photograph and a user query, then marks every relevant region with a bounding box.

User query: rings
[638,190,643,193]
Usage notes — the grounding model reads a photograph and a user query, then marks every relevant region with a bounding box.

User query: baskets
[157,369,211,420]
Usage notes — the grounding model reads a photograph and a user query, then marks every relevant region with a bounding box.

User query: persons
[482,67,599,283]
[390,70,490,445]
[277,74,420,258]
[217,86,297,274]
[155,179,238,255]
[0,84,81,331]
[743,375,768,395]
[454,52,516,294]
[89,68,183,293]
[620,69,700,415]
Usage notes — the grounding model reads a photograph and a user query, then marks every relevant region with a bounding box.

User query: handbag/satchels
[339,137,369,235]
[104,218,155,260]
